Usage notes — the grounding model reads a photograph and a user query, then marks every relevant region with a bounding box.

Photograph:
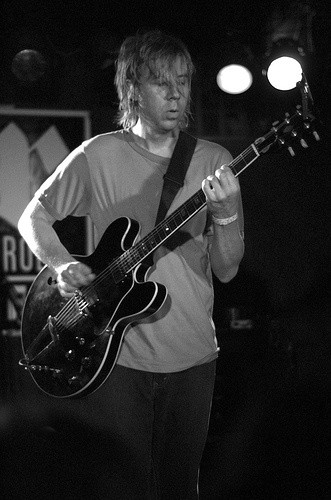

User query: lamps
[261,36,309,95]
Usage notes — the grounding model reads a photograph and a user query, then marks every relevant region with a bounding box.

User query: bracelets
[211,212,237,226]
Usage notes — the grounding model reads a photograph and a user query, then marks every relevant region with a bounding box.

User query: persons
[18,31,243,498]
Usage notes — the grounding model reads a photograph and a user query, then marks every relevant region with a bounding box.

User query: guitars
[19,100,323,400]
